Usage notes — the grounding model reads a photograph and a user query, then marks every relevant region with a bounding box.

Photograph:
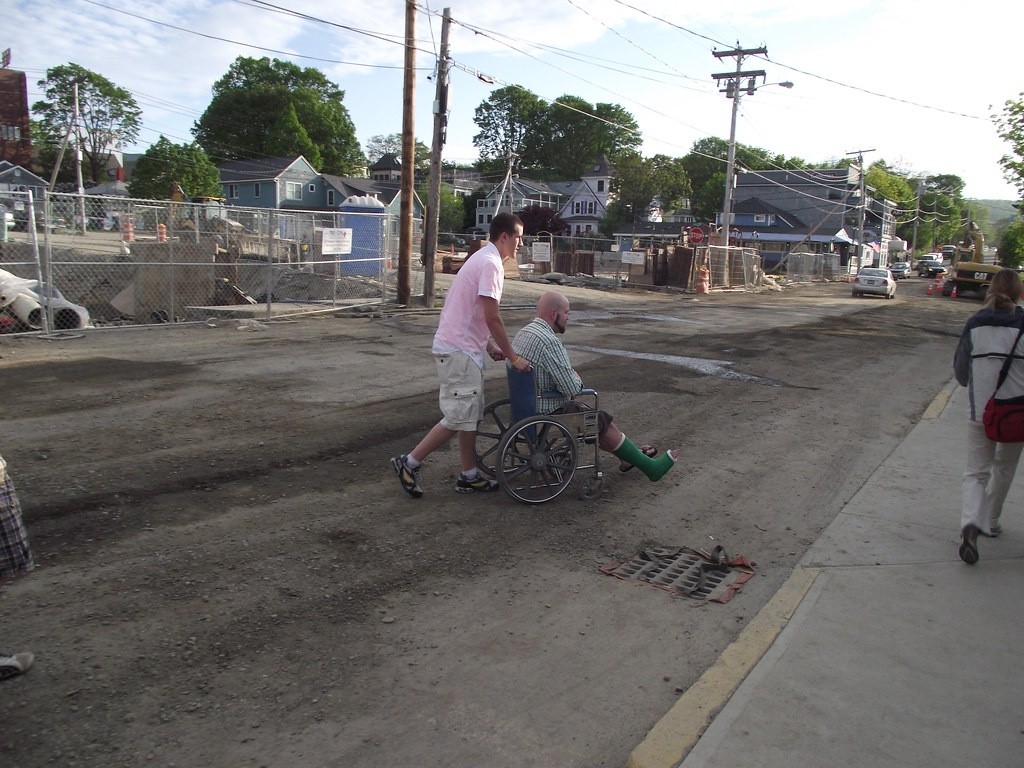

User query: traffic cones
[950,285,957,299]
[928,283,933,295]
[937,281,942,292]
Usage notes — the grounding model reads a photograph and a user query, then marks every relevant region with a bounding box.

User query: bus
[942,220,1006,302]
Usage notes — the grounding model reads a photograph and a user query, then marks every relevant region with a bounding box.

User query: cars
[0,202,15,230]
[852,268,898,300]
[917,259,945,278]
[890,262,912,280]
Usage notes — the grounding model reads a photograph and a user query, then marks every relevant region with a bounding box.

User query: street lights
[712,48,793,289]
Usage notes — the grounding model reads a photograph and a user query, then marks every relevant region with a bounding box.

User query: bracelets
[511,356,520,365]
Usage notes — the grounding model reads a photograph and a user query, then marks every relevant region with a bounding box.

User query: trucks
[921,252,942,265]
[940,245,956,258]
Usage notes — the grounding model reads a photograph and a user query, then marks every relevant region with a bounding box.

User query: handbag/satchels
[983,395,1024,443]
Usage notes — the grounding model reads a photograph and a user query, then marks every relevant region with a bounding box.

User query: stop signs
[689,227,704,246]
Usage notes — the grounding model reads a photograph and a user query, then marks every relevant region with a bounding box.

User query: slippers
[619,446,657,472]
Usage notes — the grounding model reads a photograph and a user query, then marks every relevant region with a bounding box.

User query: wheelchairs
[475,354,607,505]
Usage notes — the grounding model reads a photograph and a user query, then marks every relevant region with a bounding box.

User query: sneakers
[986,524,1002,536]
[454,472,499,494]
[389,454,423,497]
[958,524,979,564]
[0,652,34,679]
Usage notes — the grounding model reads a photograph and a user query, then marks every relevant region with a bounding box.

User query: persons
[90,211,107,218]
[511,291,680,482]
[953,267,1024,564]
[390,212,533,498]
[293,235,311,262]
